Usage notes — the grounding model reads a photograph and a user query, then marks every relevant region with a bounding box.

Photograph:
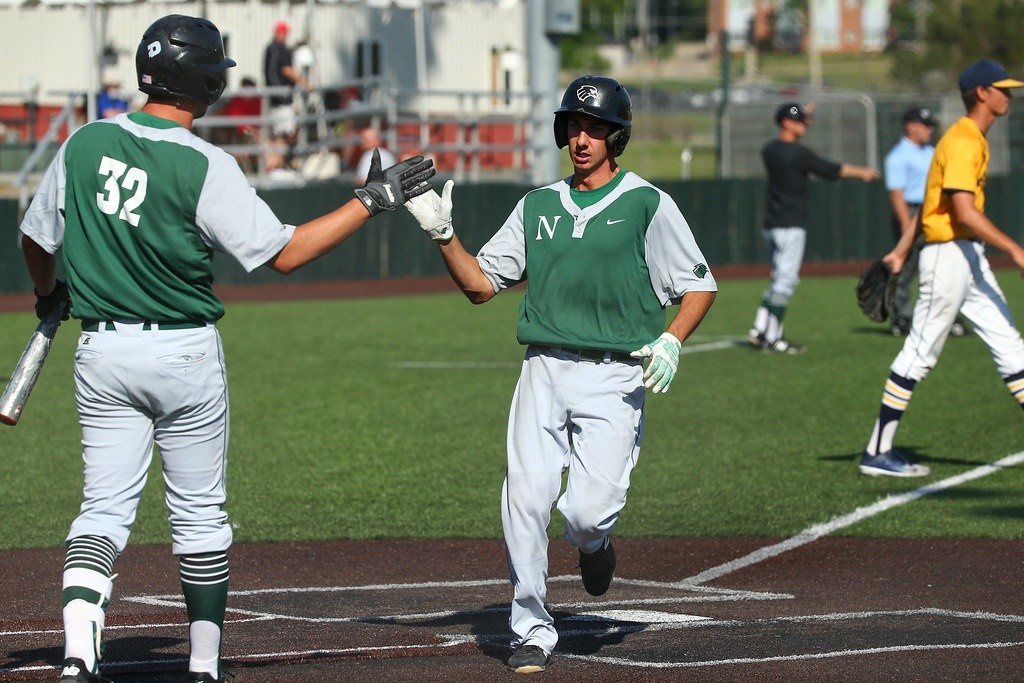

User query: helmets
[136,14,237,106]
[554,75,633,157]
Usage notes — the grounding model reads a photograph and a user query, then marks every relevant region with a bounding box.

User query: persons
[401,75,717,673]
[224,21,396,189]
[856,59,1024,478]
[88,64,127,120]
[884,107,964,336]
[19,12,436,682]
[748,103,881,355]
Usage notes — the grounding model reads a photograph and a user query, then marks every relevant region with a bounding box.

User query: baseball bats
[0,297,68,426]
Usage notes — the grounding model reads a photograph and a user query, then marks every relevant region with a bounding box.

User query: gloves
[630,332,682,393]
[34,278,70,321]
[402,179,454,241]
[353,146,436,217]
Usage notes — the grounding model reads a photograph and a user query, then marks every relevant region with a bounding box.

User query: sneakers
[576,534,616,596]
[508,646,551,673]
[859,449,929,477]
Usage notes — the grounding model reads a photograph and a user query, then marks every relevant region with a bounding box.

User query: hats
[904,108,937,125]
[959,60,1024,88]
[775,104,811,120]
[276,22,289,33]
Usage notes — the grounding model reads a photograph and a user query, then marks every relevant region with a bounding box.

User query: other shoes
[57,657,113,683]
[762,339,808,355]
[746,328,765,346]
[189,667,235,683]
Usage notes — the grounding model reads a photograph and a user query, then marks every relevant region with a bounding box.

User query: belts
[81,318,206,330]
[562,347,640,361]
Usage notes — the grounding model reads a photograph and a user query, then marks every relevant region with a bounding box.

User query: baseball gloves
[855,260,901,323]
